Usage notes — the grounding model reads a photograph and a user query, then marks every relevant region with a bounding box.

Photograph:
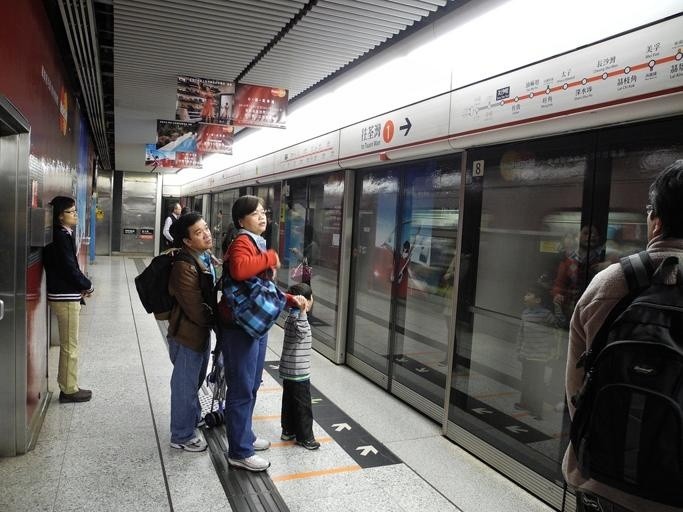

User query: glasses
[644,204,653,213]
[57,209,80,213]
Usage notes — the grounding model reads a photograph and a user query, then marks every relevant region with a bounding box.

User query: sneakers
[513,403,527,409]
[280,432,296,440]
[170,435,208,451]
[528,408,540,414]
[296,437,320,449]
[253,437,270,450]
[554,403,566,413]
[437,359,449,367]
[228,454,272,472]
[197,417,210,430]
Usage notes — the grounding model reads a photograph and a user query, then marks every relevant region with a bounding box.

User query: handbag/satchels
[218,273,286,338]
[291,258,313,282]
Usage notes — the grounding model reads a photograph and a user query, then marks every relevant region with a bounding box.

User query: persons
[514,283,562,416]
[220,102,229,121]
[166,212,218,453]
[218,195,281,473]
[213,209,224,261]
[288,226,319,323]
[552,225,620,414]
[181,207,192,215]
[562,159,683,512]
[437,244,457,367]
[44,196,94,404]
[162,203,182,250]
[197,85,217,124]
[402,241,411,267]
[279,283,320,451]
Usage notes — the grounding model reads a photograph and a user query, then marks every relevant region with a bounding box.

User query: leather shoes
[59,390,90,402]
[78,389,92,397]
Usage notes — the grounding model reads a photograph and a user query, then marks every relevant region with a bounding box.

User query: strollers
[204,304,228,427]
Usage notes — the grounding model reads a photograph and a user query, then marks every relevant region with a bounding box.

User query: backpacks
[569,250,683,507]
[135,250,205,313]
[217,233,287,341]
